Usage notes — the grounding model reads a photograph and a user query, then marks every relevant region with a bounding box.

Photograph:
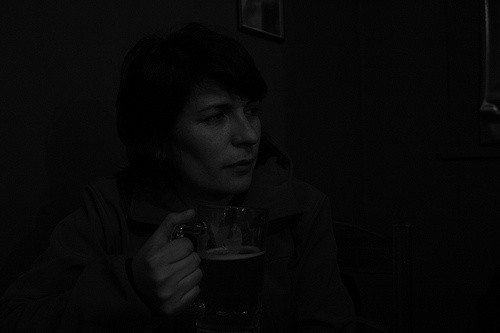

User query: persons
[0,17,358,333]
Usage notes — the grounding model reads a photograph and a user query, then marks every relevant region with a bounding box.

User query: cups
[168,206,267,333]
